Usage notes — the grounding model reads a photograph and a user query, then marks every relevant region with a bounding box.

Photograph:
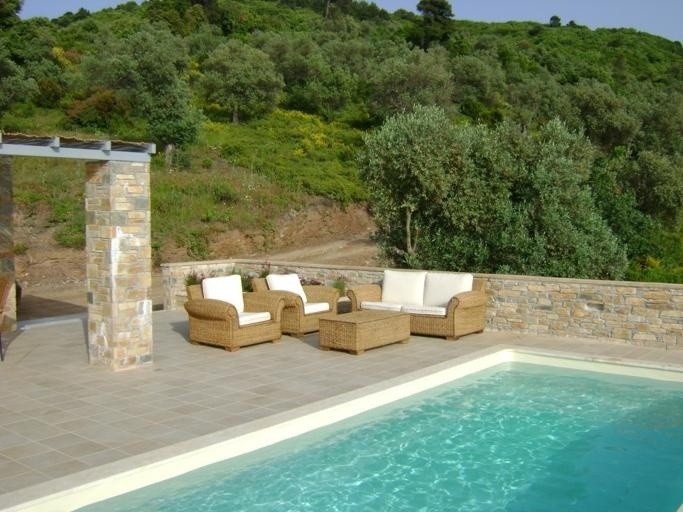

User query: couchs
[346,269,489,342]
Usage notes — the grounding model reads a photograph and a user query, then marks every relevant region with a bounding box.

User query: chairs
[183,271,341,352]
